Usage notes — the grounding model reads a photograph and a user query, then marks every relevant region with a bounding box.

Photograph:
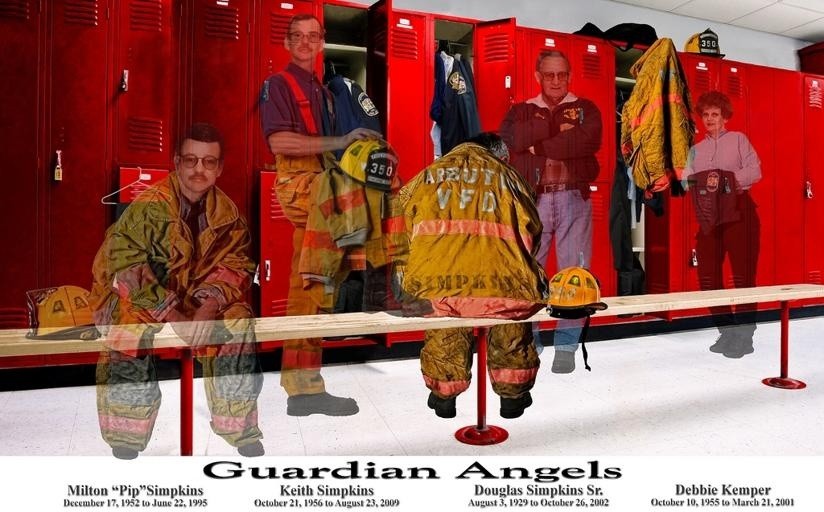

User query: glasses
[288,31,323,43]
[538,71,569,81]
[177,153,220,170]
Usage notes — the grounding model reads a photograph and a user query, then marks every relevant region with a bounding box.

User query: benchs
[0,284,824,456]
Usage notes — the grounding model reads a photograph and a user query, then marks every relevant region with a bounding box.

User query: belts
[538,182,580,194]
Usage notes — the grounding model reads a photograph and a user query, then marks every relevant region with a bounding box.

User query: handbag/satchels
[572,22,657,46]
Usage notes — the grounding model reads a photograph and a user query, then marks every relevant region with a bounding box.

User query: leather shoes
[552,350,575,373]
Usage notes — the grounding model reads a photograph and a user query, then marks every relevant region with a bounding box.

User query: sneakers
[237,441,265,456]
[113,447,139,459]
[428,392,456,418]
[287,392,359,416]
[724,335,754,358]
[365,283,434,317]
[710,331,736,353]
[500,391,532,418]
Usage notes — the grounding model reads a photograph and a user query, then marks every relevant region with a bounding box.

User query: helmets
[26,284,101,341]
[684,28,725,58]
[338,136,401,191]
[546,266,608,319]
